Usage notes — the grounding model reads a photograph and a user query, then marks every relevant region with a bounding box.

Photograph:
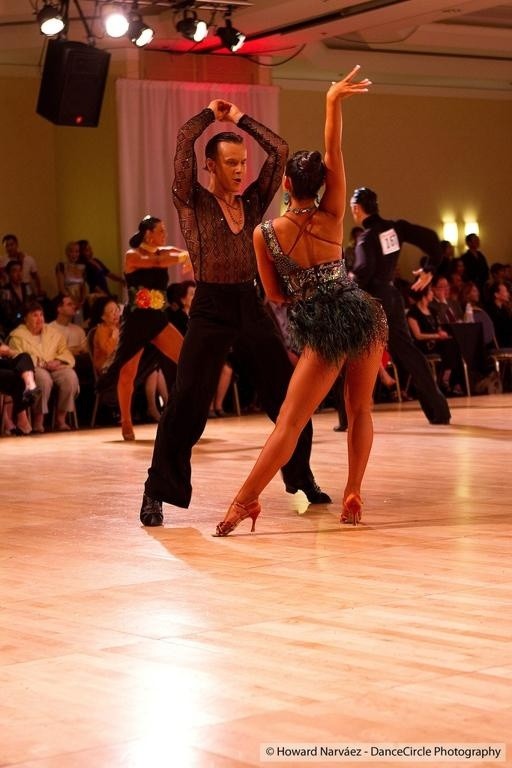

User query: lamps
[35,0,248,53]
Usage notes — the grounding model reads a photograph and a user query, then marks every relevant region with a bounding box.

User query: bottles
[463,301,474,322]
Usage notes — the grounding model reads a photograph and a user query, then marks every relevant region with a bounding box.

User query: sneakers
[334,424,348,432]
[206,408,227,419]
[139,479,164,527]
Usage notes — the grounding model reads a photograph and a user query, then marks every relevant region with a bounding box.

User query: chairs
[0,310,512,437]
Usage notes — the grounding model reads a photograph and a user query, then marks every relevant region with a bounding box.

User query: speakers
[35,41,112,129]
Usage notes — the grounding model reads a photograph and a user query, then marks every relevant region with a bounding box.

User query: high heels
[339,493,363,527]
[210,496,261,538]
[121,419,136,442]
[284,475,333,505]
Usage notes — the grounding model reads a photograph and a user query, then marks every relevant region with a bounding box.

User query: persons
[210,65,389,538]
[140,98,332,526]
